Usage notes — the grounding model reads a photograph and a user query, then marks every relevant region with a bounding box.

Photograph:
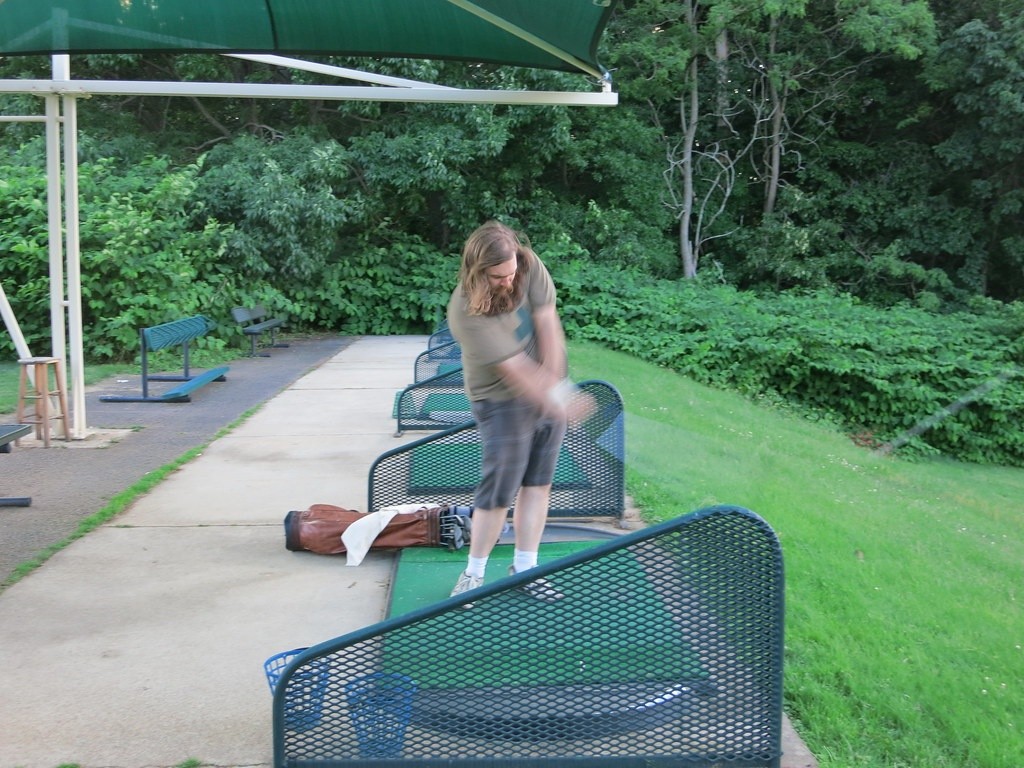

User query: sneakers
[508,563,564,604]
[451,570,484,613]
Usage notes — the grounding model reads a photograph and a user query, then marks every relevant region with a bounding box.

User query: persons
[446,222,598,610]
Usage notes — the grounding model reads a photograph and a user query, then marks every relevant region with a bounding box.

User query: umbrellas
[0,1,640,454]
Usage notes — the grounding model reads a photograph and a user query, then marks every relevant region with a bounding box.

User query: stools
[15,357,70,447]
